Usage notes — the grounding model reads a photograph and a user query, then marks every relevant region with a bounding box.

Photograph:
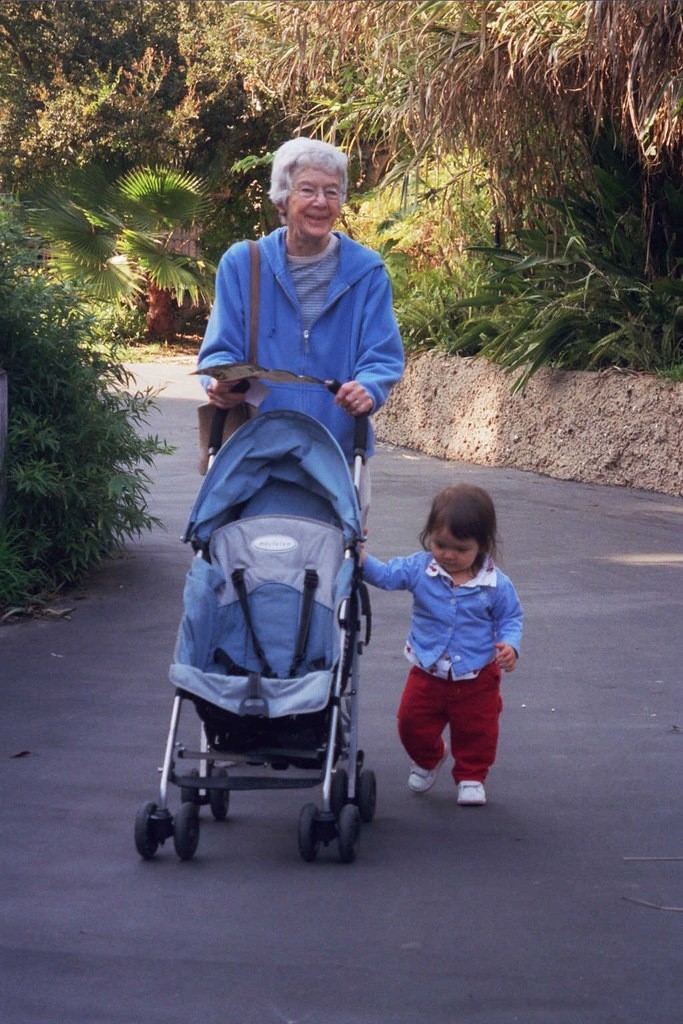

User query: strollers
[130,380,379,866]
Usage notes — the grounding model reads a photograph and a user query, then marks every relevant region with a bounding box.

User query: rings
[356,401,359,404]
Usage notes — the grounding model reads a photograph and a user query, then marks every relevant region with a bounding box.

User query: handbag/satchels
[195,391,261,474]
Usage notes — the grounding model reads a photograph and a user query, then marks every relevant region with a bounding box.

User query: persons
[361,484,524,804]
[197,136,405,766]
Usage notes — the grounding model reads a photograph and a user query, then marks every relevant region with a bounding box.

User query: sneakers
[458,781,487,805]
[407,745,449,792]
